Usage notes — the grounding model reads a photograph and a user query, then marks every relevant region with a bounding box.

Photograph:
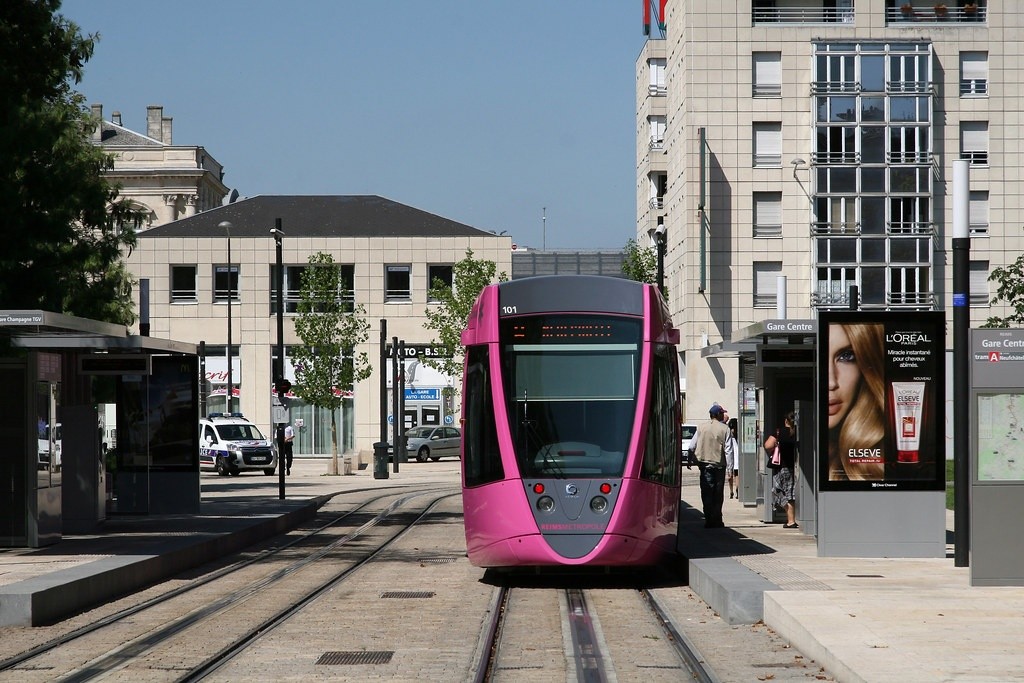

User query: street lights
[218,220,233,416]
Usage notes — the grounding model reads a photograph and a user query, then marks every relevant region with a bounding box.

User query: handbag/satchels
[771,428,781,465]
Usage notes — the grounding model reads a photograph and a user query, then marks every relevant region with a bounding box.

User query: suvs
[200,411,277,475]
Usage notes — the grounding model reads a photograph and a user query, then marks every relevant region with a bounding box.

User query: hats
[709,405,727,415]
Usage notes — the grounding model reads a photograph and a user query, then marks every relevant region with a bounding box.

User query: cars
[386,424,460,461]
[37,423,62,472]
[681,425,699,465]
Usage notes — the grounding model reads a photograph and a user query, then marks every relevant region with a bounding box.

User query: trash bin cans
[398,436,409,462]
[372,441,390,479]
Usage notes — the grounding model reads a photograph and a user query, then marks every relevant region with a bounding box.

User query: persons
[687,405,734,530]
[721,416,738,499]
[764,412,798,528]
[275,423,295,475]
[830,324,884,480]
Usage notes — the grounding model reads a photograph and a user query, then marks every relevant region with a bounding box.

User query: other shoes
[286,468,291,475]
[783,522,799,528]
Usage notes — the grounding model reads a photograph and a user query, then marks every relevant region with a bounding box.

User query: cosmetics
[891,382,925,464]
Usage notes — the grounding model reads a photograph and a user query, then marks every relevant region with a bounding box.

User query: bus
[460,275,683,570]
